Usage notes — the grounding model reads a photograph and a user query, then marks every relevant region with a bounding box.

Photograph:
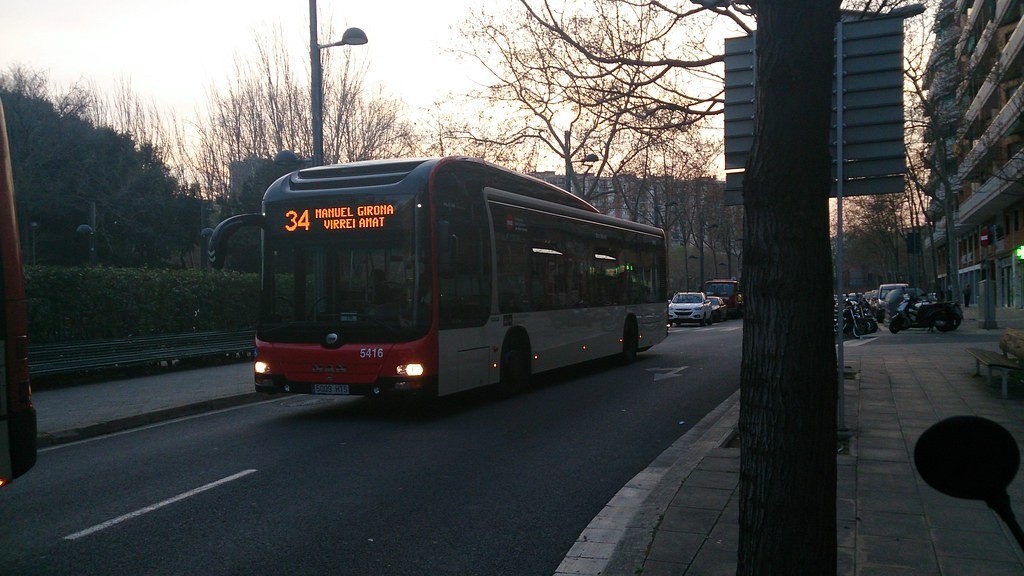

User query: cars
[668,291,713,327]
[706,296,727,322]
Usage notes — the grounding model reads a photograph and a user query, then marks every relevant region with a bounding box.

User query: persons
[963,285,971,308]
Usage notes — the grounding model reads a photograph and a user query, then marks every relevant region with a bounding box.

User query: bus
[206,154,669,420]
[0,93,39,493]
[705,280,743,318]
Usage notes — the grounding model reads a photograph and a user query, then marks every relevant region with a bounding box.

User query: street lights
[838,1,930,443]
[565,129,598,194]
[308,0,370,174]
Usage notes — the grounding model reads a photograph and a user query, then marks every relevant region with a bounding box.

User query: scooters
[888,286,963,333]
[834,289,878,339]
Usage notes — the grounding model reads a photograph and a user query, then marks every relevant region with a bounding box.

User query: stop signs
[979,226,989,246]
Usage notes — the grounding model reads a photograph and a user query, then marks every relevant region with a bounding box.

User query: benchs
[966,328,1024,398]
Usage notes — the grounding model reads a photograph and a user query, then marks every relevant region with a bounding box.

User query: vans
[877,283,910,323]
